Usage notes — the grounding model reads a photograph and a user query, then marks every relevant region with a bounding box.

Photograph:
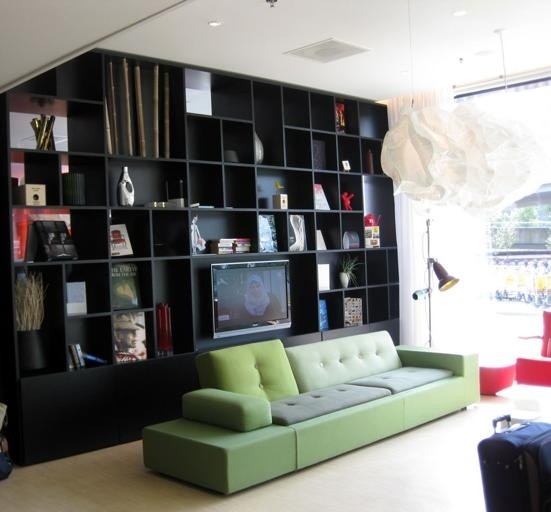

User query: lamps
[412,219,459,349]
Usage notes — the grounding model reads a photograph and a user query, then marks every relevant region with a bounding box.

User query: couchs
[140,327,481,498]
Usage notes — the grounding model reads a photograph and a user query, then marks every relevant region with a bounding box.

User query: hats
[112,312,144,330]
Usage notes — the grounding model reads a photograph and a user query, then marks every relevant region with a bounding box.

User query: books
[320,299,329,331]
[12,207,79,261]
[259,213,279,252]
[315,183,330,210]
[113,311,148,364]
[66,281,87,314]
[365,226,381,248]
[68,344,108,369]
[288,214,308,250]
[156,302,175,358]
[345,297,362,327]
[111,263,141,310]
[211,238,252,255]
[110,224,134,257]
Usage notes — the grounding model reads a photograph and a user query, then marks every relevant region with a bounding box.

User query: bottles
[364,143,375,175]
[119,165,135,207]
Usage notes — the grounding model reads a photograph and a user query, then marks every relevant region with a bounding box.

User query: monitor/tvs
[210,260,292,340]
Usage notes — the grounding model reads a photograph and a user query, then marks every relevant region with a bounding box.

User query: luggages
[477,413,551,511]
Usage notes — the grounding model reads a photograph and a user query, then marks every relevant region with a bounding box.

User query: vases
[17,329,57,373]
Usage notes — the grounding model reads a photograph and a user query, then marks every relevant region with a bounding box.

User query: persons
[191,215,205,255]
[241,274,282,323]
[115,312,141,362]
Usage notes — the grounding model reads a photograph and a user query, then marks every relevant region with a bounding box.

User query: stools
[480,354,551,396]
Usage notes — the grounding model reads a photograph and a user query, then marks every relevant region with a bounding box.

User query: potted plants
[338,253,365,288]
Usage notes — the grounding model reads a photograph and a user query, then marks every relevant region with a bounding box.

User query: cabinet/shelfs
[0,46,402,467]
